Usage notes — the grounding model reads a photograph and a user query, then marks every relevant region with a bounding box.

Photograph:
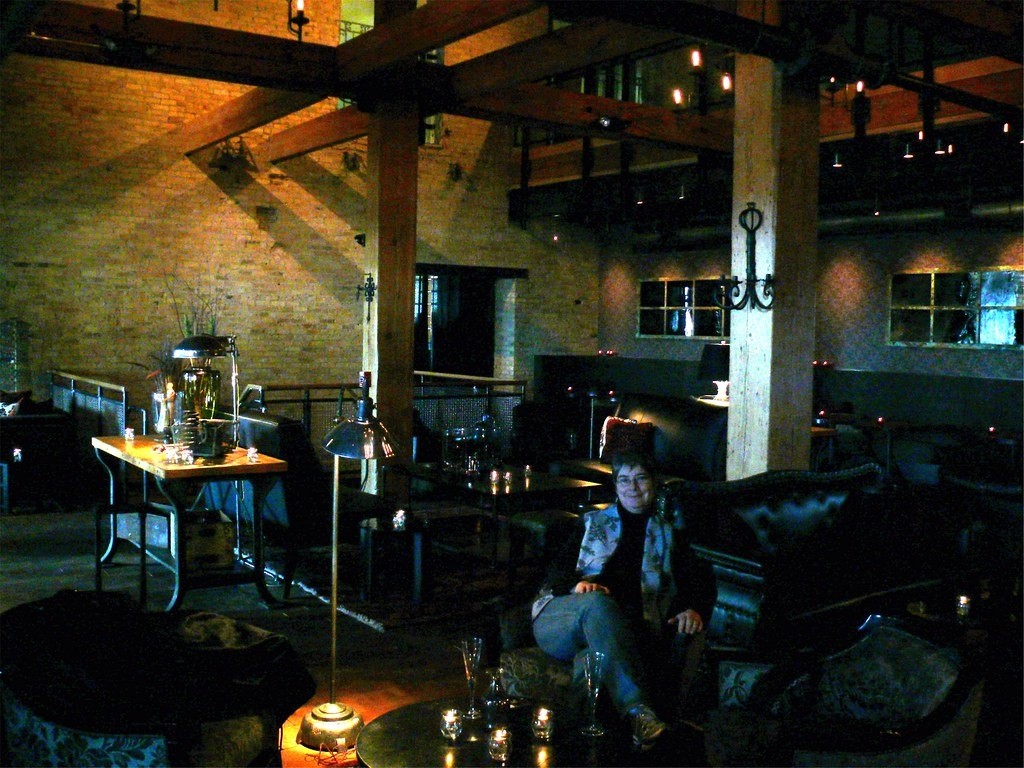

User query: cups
[392,513,406,532]
[181,449,194,465]
[488,730,512,761]
[439,708,463,740]
[489,469,499,486]
[523,465,532,474]
[531,709,554,740]
[248,449,258,463]
[956,596,970,615]
[502,472,512,485]
[124,429,134,441]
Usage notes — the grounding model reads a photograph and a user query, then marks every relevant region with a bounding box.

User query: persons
[529,447,719,755]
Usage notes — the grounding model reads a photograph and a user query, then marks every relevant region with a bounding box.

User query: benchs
[565,392,729,502]
[199,404,388,599]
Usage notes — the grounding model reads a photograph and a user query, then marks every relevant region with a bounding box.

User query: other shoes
[633,712,667,750]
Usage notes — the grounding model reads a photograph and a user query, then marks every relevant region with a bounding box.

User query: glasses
[616,476,652,486]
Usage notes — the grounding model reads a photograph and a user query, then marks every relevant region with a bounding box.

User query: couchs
[0,590,317,768]
[770,626,985,767]
[484,517,718,725]
[662,463,1024,664]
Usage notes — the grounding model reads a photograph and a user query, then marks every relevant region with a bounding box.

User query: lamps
[833,153,843,169]
[288,0,309,41]
[711,202,776,310]
[935,140,945,157]
[300,371,409,752]
[356,273,376,321]
[903,143,916,159]
[173,333,241,453]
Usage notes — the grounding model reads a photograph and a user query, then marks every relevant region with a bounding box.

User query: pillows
[600,417,653,467]
[646,426,682,466]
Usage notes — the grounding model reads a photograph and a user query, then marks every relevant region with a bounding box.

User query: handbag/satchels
[165,609,289,673]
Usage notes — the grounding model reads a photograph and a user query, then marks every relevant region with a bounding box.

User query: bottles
[480,668,513,731]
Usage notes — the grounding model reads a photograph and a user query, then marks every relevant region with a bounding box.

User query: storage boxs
[170,509,235,569]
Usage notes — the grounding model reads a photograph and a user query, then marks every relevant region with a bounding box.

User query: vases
[151,391,185,434]
[178,368,221,445]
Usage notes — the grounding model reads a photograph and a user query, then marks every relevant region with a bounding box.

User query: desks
[357,693,709,768]
[809,426,839,468]
[396,457,605,570]
[0,401,78,511]
[91,434,291,612]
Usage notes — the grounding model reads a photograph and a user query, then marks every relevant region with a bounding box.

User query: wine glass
[460,637,482,720]
[580,652,606,737]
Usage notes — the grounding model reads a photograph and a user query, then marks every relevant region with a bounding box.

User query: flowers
[121,332,182,392]
